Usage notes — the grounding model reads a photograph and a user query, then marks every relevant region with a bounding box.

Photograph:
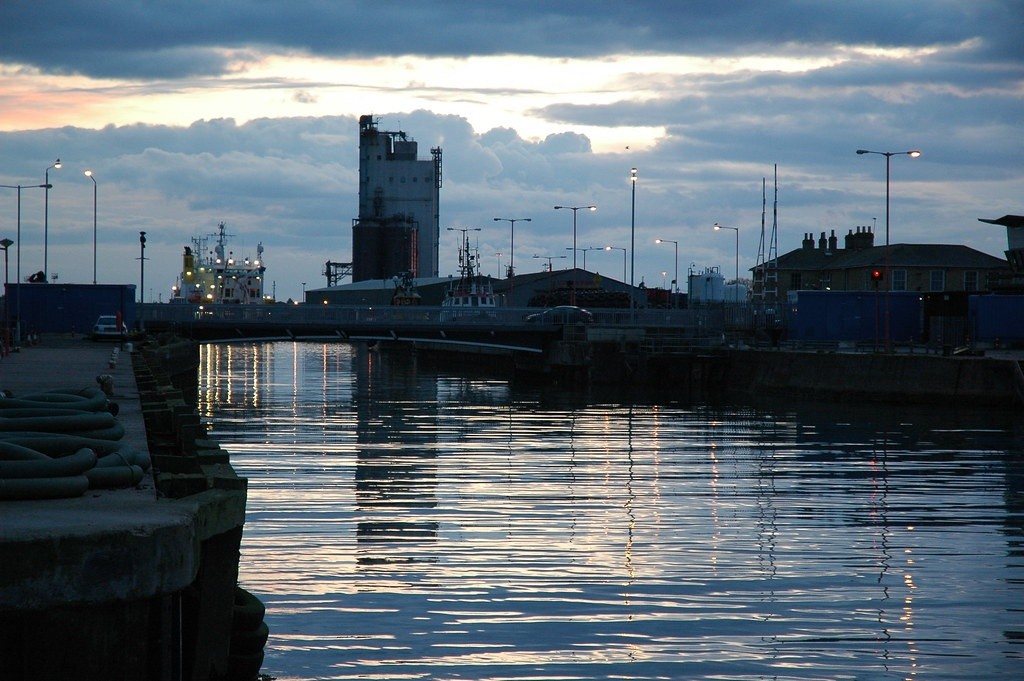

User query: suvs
[93,314,128,343]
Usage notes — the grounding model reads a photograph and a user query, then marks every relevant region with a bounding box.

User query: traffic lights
[871,270,880,281]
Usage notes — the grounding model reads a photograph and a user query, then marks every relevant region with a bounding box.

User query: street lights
[494,217,532,266]
[655,239,677,295]
[45,158,63,284]
[84,171,96,284]
[857,149,921,352]
[630,167,637,326]
[533,254,567,270]
[447,228,481,276]
[0,184,53,348]
[713,224,739,302]
[554,205,597,306]
[606,246,626,283]
[662,272,667,290]
[566,247,604,270]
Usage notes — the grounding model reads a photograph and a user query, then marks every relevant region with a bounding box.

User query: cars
[522,306,594,325]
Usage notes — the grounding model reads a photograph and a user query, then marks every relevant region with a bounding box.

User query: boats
[439,234,497,321]
[170,221,267,319]
